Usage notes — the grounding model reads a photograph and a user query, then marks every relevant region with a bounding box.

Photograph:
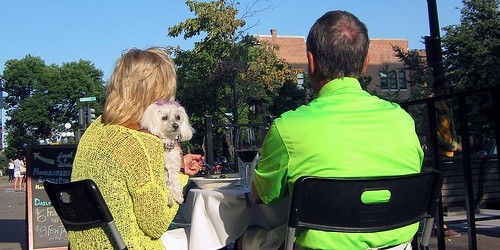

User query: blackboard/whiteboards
[26,145,87,249]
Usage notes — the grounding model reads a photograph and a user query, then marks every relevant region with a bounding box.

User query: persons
[67,46,204,250]
[7,157,27,192]
[233,10,425,250]
[420,115,463,237]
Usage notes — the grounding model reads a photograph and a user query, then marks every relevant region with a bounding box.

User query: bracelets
[180,167,185,173]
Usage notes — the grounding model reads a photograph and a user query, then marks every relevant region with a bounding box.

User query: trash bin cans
[236,150,258,190]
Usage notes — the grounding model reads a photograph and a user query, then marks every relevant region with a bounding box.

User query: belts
[377,244,400,250]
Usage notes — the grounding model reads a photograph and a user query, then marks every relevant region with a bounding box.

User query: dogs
[138,98,194,208]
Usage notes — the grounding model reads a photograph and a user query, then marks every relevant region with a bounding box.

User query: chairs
[285,170,446,250]
[43,179,128,250]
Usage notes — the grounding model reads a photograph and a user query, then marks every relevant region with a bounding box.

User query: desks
[182,189,287,250]
